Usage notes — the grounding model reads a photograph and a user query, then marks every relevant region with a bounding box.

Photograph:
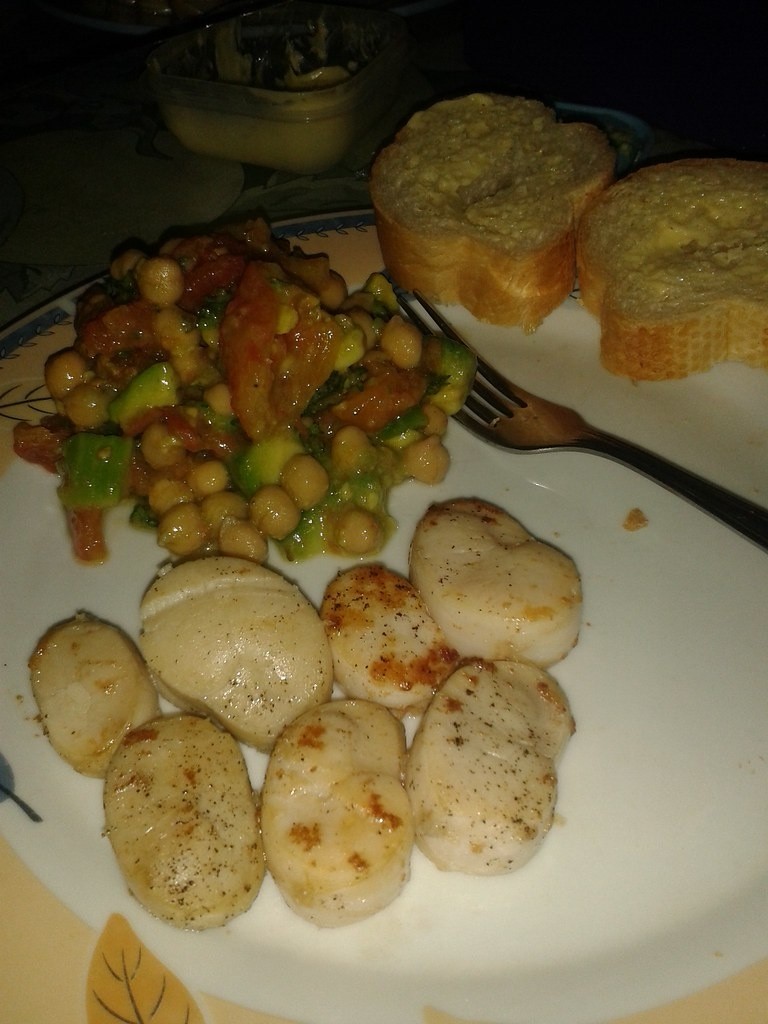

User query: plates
[345,95,653,208]
[1,208,767,1023]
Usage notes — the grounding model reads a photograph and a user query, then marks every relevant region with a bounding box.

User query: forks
[391,289,767,550]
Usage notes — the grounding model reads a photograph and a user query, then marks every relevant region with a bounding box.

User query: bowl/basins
[136,3,410,175]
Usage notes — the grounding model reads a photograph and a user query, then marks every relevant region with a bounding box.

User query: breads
[572,156,767,382]
[366,92,614,333]
